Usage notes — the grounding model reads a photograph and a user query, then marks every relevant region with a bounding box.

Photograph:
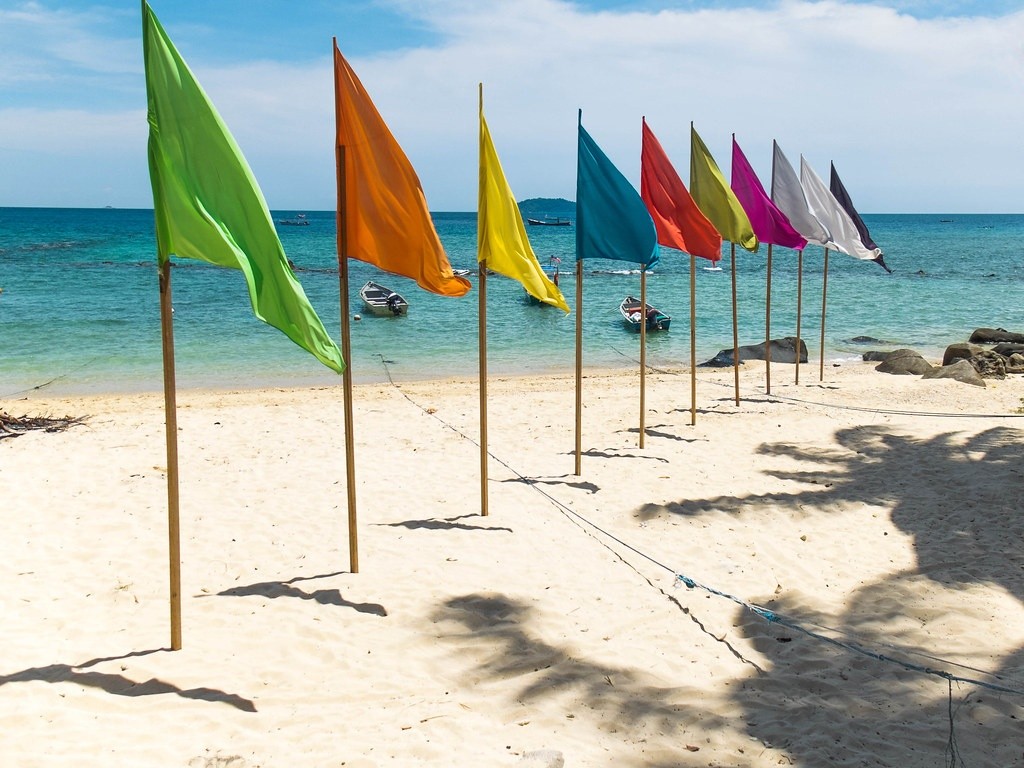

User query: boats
[278,219,311,226]
[526,218,572,226]
[620,295,672,332]
[521,255,561,305]
[358,281,410,317]
